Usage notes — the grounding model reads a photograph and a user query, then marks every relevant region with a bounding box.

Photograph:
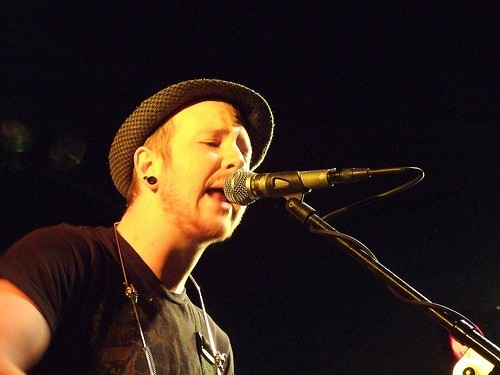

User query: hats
[109,78,274,198]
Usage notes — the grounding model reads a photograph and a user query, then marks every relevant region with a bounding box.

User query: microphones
[224,166,372,205]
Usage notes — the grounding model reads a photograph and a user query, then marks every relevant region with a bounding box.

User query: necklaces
[113,223,227,375]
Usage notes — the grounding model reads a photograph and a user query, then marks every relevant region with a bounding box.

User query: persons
[0,80,274,375]
